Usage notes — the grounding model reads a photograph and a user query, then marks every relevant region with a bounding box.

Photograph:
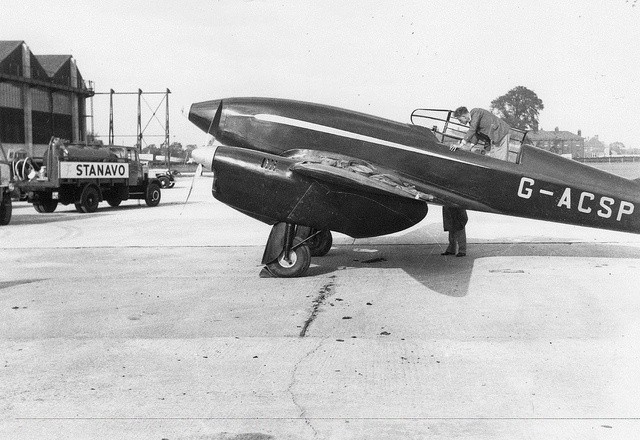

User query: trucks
[13,136,161,212]
[143,160,176,188]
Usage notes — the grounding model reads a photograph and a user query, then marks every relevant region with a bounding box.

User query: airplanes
[188,96,639,278]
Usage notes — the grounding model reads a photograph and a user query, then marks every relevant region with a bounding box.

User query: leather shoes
[441,251,454,255]
[456,252,466,257]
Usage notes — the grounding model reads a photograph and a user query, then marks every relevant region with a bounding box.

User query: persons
[449,107,512,163]
[442,205,469,258]
